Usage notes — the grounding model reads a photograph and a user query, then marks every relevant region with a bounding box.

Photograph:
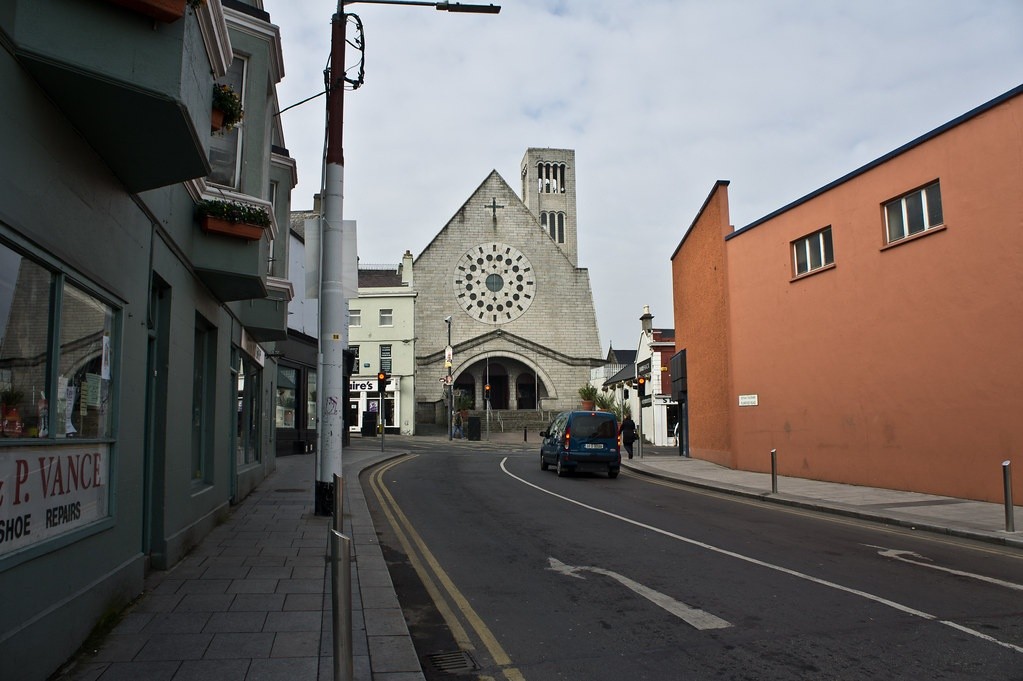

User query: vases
[212,110,226,131]
[202,214,264,240]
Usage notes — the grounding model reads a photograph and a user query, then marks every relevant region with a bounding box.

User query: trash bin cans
[468,416,481,441]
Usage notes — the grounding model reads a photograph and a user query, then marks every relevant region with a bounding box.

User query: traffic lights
[637,375,646,400]
[377,371,393,394]
[484,383,492,401]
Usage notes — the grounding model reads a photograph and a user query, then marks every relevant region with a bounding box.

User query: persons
[619,413,635,459]
[371,403,376,412]
[452,409,465,440]
[674,423,679,447]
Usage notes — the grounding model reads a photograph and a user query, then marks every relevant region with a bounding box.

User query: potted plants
[115,0,203,27]
[454,389,476,422]
[579,383,632,431]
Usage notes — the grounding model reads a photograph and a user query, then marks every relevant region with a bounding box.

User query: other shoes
[629,455,633,459]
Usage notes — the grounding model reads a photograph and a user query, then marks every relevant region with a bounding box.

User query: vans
[539,412,622,478]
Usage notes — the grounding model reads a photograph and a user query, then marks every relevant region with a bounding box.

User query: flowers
[213,82,244,137]
[196,199,272,227]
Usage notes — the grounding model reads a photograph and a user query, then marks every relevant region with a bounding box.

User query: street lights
[314,1,502,518]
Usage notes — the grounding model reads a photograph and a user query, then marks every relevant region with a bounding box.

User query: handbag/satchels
[455,420,461,426]
[633,428,639,440]
